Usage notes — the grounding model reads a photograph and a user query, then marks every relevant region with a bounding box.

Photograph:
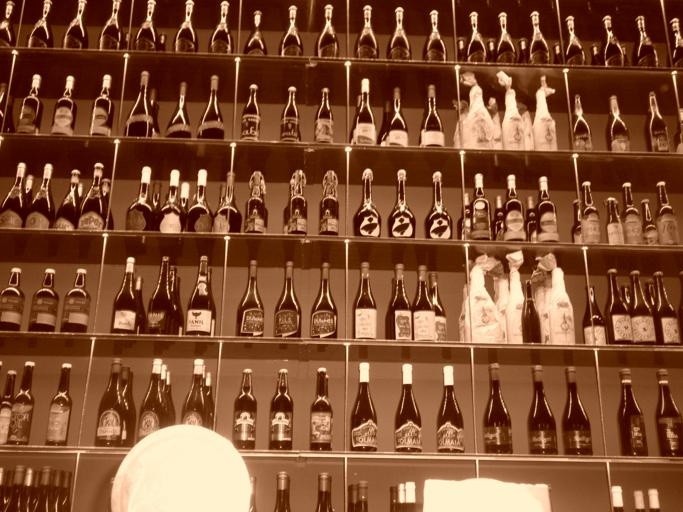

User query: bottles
[1,459,682,510]
[1,156,683,253]
[0,260,682,350]
[1,355,683,458]
[1,70,681,152]
[1,3,683,69]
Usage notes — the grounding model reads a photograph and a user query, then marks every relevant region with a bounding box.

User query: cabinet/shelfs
[0,0,683,512]
